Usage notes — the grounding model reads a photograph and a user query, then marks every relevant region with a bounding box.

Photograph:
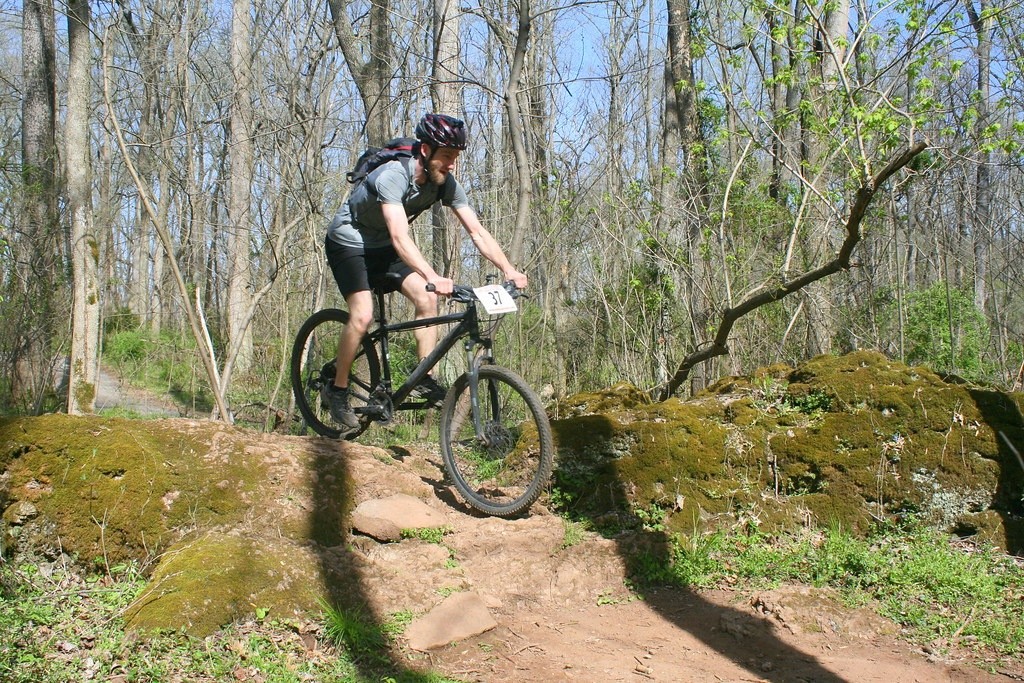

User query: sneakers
[320,379,359,428]
[409,374,447,406]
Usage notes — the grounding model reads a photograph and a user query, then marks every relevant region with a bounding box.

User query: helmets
[416,114,467,150]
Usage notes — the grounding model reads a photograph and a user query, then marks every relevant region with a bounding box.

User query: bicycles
[291,274,554,518]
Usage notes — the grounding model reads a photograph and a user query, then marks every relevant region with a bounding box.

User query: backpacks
[346,138,445,224]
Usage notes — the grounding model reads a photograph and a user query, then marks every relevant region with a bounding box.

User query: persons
[320,114,528,429]
